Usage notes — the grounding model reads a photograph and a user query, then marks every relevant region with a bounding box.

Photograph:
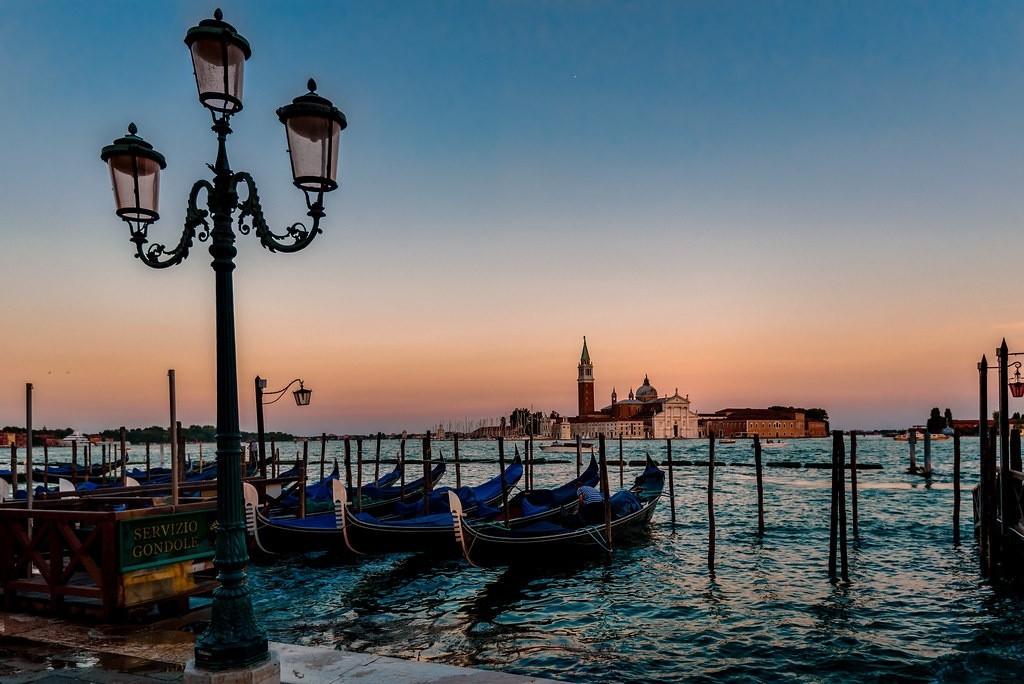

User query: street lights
[977,354,1024,577]
[255,375,313,479]
[96,6,352,669]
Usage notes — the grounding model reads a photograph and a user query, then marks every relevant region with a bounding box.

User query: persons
[251,440,257,461]
[576,481,604,518]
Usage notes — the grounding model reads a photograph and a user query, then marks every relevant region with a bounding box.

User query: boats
[893,429,950,441]
[447,450,666,572]
[240,441,528,555]
[331,447,600,557]
[539,442,600,452]
[751,439,788,448]
[55,430,94,447]
[718,440,737,443]
[0,451,449,519]
